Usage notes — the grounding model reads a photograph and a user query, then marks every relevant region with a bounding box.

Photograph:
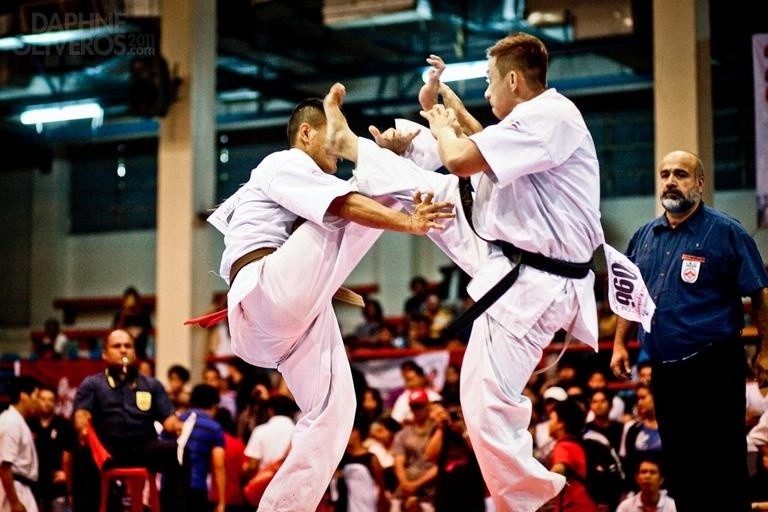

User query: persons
[203,98,455,511]
[1,286,303,512]
[519,358,676,512]
[320,275,487,512]
[746,379,768,452]
[609,152,768,511]
[322,30,655,512]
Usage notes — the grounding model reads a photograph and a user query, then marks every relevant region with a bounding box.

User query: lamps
[100,468,161,511]
[20,97,104,126]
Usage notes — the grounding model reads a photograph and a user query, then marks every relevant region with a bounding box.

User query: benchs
[12,283,767,390]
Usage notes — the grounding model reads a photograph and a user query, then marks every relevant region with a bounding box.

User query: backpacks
[565,429,627,505]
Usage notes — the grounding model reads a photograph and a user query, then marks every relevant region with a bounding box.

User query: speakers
[128,53,170,118]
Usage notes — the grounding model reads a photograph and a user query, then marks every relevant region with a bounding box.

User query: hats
[544,385,568,402]
[408,388,428,406]
